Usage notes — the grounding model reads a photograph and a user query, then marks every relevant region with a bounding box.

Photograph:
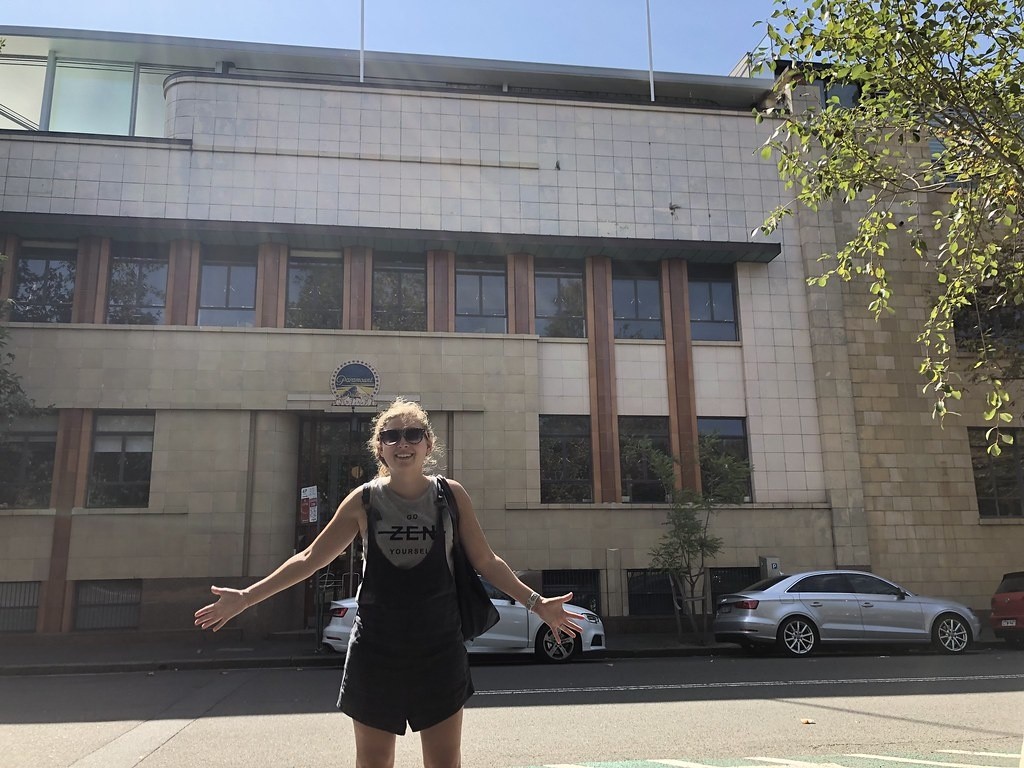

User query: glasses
[378,427,431,445]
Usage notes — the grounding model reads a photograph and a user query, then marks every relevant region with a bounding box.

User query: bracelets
[525,591,541,611]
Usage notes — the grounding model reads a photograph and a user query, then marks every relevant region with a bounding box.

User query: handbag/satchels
[437,475,500,640]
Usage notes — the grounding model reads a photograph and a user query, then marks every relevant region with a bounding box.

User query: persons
[195,396,587,768]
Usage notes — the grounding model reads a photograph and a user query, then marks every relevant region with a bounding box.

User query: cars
[322,573,606,665]
[990,570,1024,647]
[714,570,982,657]
